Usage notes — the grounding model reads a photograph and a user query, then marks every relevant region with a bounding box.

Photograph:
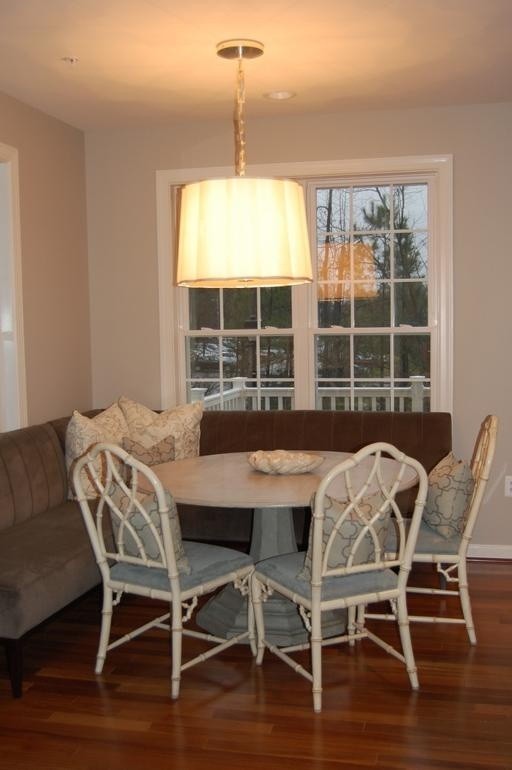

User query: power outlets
[504,476,512,498]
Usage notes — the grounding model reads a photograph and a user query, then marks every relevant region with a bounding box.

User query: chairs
[359,416,497,641]
[251,439,424,712]
[72,445,255,696]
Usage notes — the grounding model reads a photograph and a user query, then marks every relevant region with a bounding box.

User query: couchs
[1,411,452,695]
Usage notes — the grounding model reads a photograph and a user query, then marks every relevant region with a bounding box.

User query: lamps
[174,35,312,291]
[314,240,381,302]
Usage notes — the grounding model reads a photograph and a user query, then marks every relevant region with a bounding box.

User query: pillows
[423,453,470,541]
[297,480,398,583]
[122,436,178,482]
[106,484,190,575]
[120,399,204,462]
[67,405,128,502]
